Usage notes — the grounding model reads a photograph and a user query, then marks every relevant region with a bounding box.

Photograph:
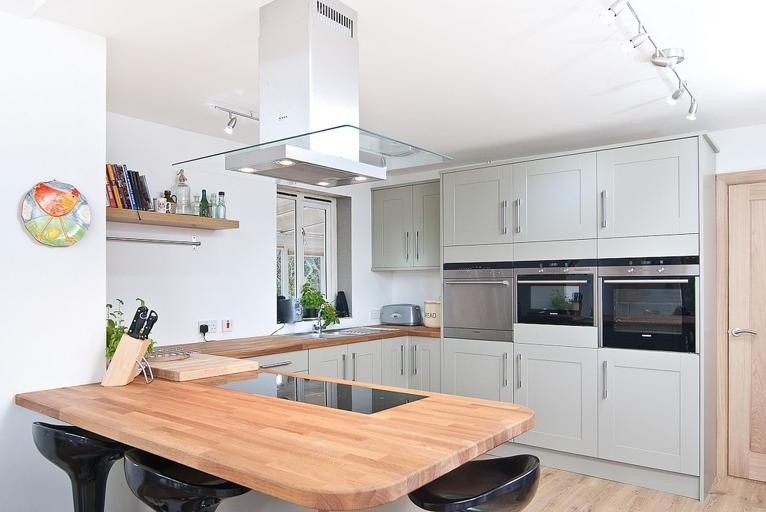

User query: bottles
[155,169,225,218]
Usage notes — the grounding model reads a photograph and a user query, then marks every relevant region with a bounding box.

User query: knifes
[124,305,158,339]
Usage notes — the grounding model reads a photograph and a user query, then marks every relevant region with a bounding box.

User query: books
[106,161,151,214]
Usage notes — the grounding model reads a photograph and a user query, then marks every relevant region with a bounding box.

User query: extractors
[170,0,454,191]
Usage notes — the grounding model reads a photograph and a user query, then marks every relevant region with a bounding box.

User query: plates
[19,181,90,247]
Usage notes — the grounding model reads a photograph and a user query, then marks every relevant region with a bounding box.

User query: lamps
[597,0,699,122]
[222,112,239,134]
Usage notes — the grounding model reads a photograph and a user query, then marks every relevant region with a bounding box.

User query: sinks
[279,326,400,339]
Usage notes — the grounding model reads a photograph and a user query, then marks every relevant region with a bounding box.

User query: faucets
[312,304,326,338]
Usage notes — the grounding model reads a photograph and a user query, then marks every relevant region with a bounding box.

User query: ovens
[443,261,512,343]
[512,260,594,326]
[596,256,699,351]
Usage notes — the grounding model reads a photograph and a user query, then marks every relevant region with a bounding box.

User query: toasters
[380,304,421,326]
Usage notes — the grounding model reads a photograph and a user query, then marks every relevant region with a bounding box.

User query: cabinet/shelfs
[440,134,720,259]
[372,177,441,269]
[260,337,409,393]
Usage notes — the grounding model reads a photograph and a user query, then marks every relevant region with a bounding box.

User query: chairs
[411,451,540,512]
[124,450,249,512]
[31,420,124,512]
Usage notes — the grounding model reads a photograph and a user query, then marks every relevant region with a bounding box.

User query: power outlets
[198,321,216,333]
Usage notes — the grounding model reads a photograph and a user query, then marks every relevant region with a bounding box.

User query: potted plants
[302,283,340,327]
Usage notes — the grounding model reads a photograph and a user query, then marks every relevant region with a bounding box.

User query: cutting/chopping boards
[142,351,259,383]
